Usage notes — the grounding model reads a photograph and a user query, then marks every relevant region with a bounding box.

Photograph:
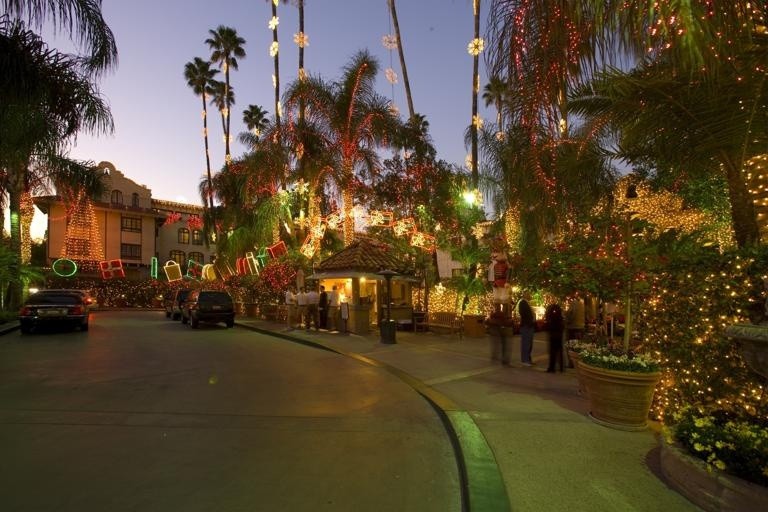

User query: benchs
[412,307,466,341]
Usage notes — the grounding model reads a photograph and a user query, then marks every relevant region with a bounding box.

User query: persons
[519,291,585,374]
[285,285,340,332]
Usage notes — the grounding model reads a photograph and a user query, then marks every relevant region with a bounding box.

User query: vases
[235,269,265,316]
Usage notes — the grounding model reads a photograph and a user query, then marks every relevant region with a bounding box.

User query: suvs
[181,290,235,329]
[164,290,194,320]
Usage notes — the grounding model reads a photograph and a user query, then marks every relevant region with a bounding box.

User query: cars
[19,290,93,334]
[71,290,99,310]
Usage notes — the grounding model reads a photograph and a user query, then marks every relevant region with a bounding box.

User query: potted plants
[260,294,280,320]
[574,345,662,433]
